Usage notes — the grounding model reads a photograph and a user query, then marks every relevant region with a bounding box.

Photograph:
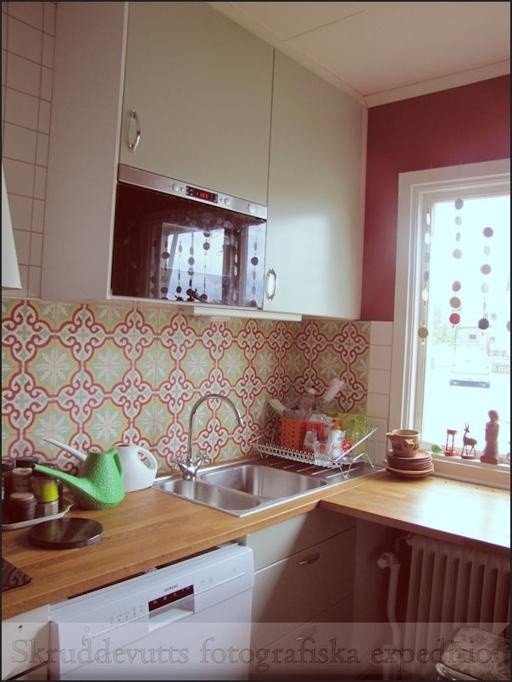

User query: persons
[460,419,478,459]
[483,408,501,460]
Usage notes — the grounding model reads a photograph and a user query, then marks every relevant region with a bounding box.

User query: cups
[386,428,419,457]
[313,441,334,466]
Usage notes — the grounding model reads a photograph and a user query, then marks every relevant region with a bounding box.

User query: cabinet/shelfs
[245,509,356,682]
[263,2,368,321]
[117,0,277,209]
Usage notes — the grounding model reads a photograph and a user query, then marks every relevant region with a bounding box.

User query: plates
[383,450,435,479]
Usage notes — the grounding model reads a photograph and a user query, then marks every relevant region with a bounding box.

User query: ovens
[50,545,252,682]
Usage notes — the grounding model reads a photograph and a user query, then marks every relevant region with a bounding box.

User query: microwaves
[107,166,266,311]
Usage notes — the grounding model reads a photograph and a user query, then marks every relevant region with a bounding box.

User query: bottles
[0,455,59,521]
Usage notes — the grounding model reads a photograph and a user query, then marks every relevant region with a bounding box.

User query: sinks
[149,472,261,518]
[200,458,327,500]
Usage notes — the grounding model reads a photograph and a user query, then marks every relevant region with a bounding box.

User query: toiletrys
[329,416,343,458]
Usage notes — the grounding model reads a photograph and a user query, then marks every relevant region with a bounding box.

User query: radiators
[401,538,508,682]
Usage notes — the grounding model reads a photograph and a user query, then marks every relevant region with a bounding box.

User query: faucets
[178,393,244,476]
